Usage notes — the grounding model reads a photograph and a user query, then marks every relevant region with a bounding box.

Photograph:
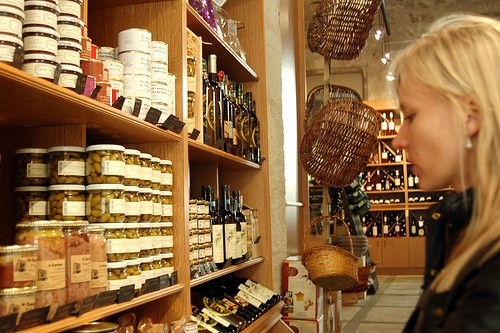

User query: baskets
[299,100,382,188]
[302,84,363,134]
[307,0,381,61]
[301,216,359,292]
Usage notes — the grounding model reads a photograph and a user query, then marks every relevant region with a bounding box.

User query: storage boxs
[281,255,379,333]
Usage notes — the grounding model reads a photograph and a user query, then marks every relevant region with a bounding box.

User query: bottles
[409,193,444,203]
[328,168,406,237]
[414,173,420,189]
[369,139,403,164]
[418,216,425,236]
[411,220,417,236]
[388,112,395,135]
[408,169,414,189]
[190,273,281,333]
[200,183,260,271]
[381,112,388,136]
[200,53,261,165]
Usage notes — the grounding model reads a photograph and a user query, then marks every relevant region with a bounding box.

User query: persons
[391,13,500,333]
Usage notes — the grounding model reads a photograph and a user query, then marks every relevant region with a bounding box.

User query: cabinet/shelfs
[0,0,297,333]
[309,98,456,275]
